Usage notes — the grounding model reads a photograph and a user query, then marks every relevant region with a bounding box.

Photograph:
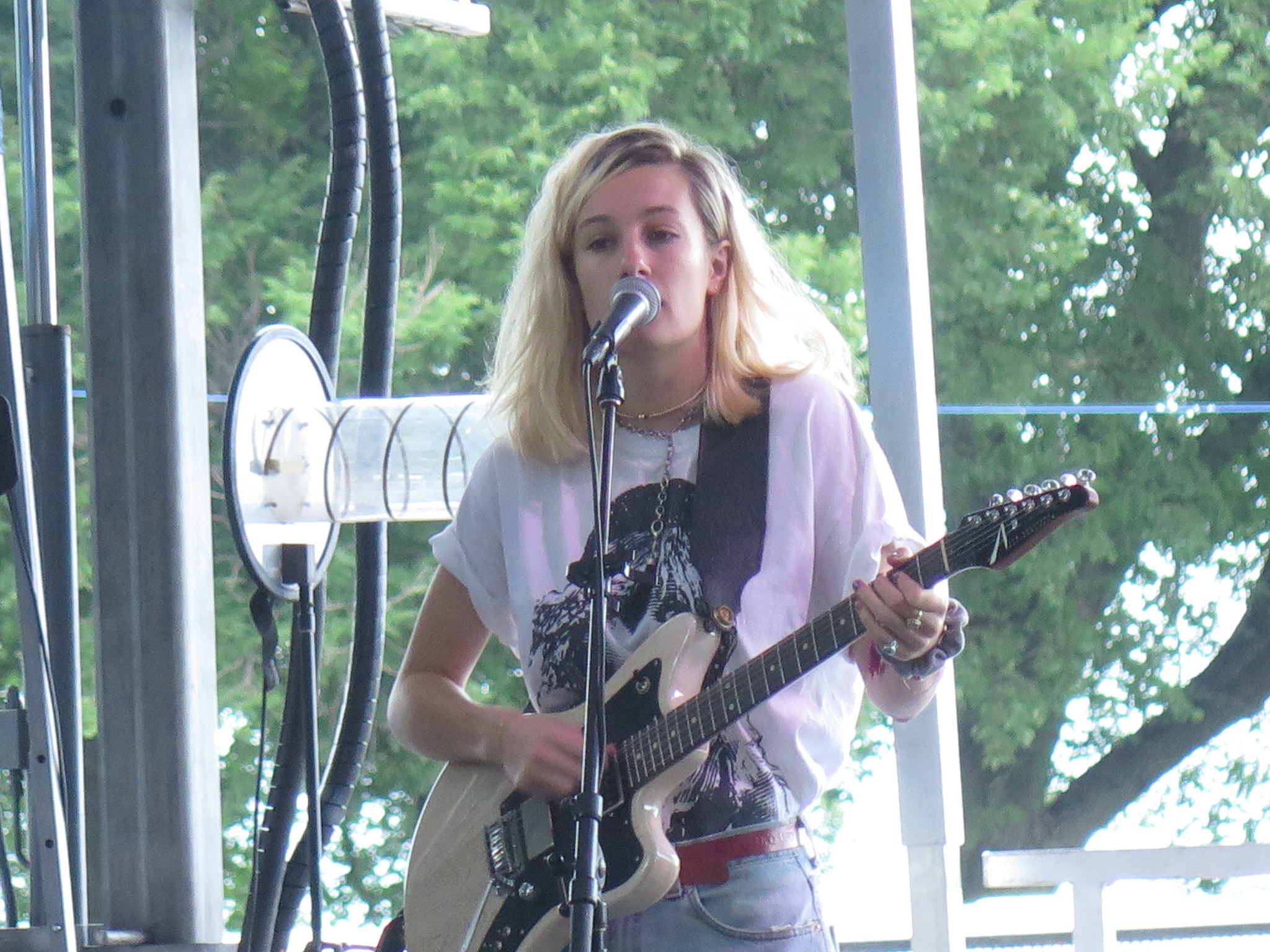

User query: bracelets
[867,598,971,695]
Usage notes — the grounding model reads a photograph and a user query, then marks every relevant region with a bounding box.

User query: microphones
[581,275,660,368]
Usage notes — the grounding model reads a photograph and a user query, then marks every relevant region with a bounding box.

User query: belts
[675,822,807,885]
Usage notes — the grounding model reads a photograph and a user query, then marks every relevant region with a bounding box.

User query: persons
[385,123,940,952]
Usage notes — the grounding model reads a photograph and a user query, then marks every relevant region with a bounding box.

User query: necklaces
[615,374,708,569]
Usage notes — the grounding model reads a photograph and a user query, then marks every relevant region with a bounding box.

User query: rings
[879,637,900,657]
[903,611,924,630]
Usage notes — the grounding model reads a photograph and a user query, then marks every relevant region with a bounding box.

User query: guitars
[401,467,1100,952]
